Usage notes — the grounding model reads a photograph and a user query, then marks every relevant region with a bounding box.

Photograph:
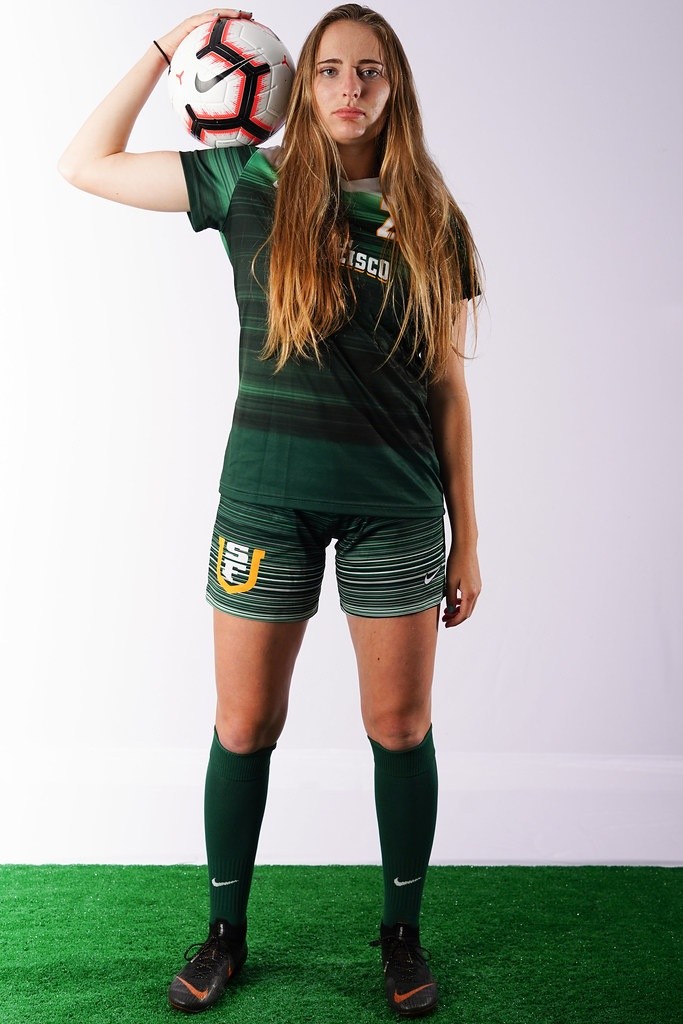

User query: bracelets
[153,41,171,67]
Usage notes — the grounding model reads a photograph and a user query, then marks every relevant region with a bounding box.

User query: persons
[62,2,484,1020]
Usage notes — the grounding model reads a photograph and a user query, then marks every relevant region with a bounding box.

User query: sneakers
[168,918,248,1012]
[378,920,440,1016]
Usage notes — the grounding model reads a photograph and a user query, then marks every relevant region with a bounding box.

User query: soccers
[170,15,296,149]
